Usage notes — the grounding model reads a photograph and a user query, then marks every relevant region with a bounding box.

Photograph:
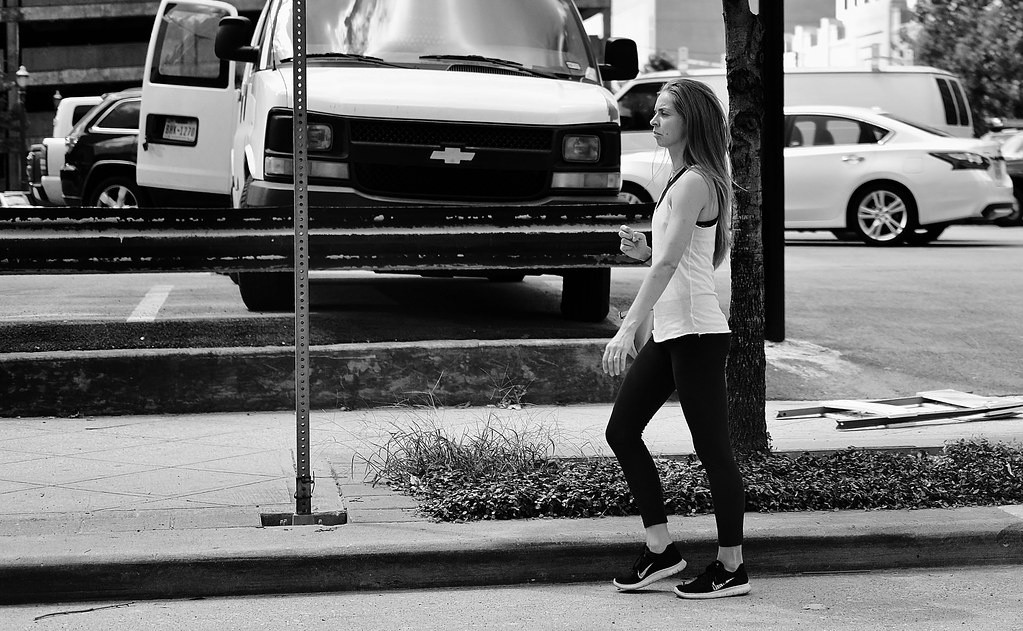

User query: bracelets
[640,254,652,264]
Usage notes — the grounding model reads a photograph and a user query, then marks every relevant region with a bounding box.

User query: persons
[602,78,752,599]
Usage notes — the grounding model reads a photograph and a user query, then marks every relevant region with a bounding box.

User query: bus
[613,66,976,156]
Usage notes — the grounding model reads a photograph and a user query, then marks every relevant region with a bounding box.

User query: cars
[623,104,1019,244]
[978,127,1021,203]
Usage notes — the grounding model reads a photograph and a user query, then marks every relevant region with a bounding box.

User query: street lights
[15,66,29,191]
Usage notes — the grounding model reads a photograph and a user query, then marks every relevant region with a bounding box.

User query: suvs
[24,87,143,208]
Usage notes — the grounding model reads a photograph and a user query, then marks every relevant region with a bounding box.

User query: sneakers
[613,541,687,590]
[673,559,751,599]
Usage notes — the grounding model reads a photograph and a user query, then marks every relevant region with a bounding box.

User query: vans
[135,0,638,325]
